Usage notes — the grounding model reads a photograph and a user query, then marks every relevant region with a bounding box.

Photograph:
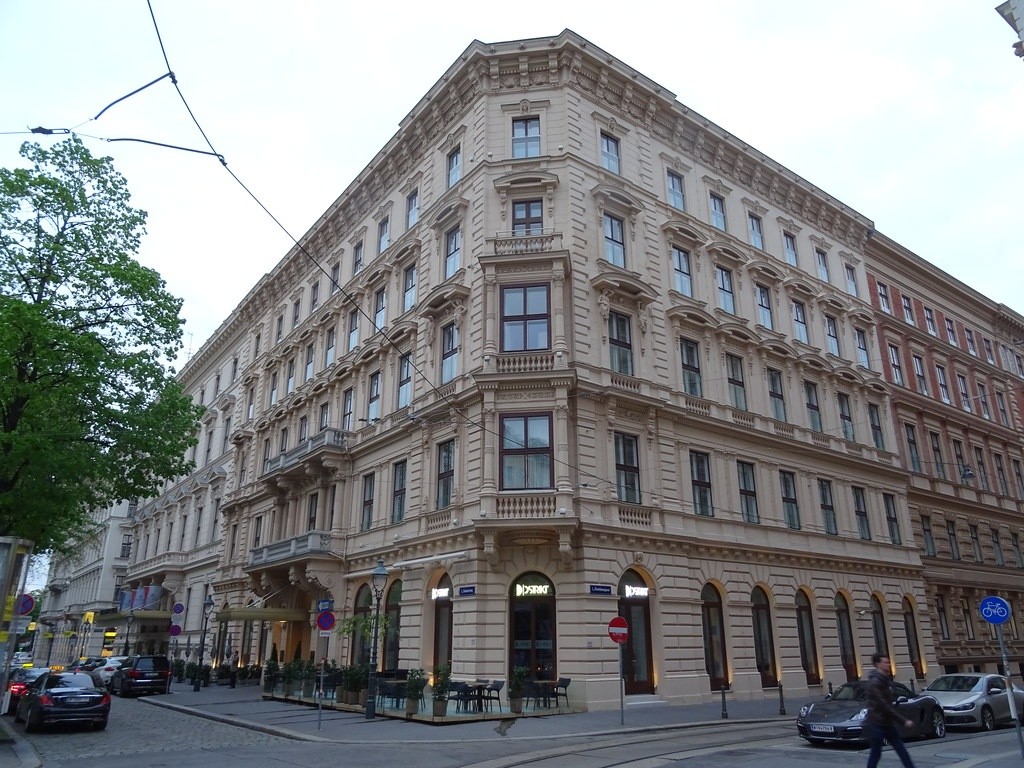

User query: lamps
[912,460,975,479]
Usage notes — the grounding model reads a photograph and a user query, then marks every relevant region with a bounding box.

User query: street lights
[193,594,215,692]
[365,560,390,719]
[80,618,91,657]
[123,610,135,655]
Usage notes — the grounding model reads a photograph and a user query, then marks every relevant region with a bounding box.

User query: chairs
[520,679,540,709]
[377,672,425,712]
[448,679,505,713]
[314,671,341,700]
[465,681,493,713]
[548,678,571,708]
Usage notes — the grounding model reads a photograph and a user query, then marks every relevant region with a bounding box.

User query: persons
[228,650,239,688]
[865,652,916,768]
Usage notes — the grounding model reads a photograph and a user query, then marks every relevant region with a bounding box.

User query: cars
[12,652,33,665]
[921,672,1024,731]
[14,670,111,732]
[67,655,127,688]
[6,667,50,712]
[110,655,173,696]
[797,680,946,748]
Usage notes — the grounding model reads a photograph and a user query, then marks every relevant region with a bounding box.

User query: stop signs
[608,617,629,643]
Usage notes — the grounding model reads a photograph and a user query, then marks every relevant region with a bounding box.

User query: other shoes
[227,686,234,689]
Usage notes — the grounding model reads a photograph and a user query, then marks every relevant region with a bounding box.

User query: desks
[383,680,408,708]
[534,680,559,707]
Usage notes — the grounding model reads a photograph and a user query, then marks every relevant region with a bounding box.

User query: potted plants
[509,667,531,712]
[405,668,429,714]
[335,665,367,706]
[172,659,261,687]
[432,659,452,716]
[263,659,315,697]
